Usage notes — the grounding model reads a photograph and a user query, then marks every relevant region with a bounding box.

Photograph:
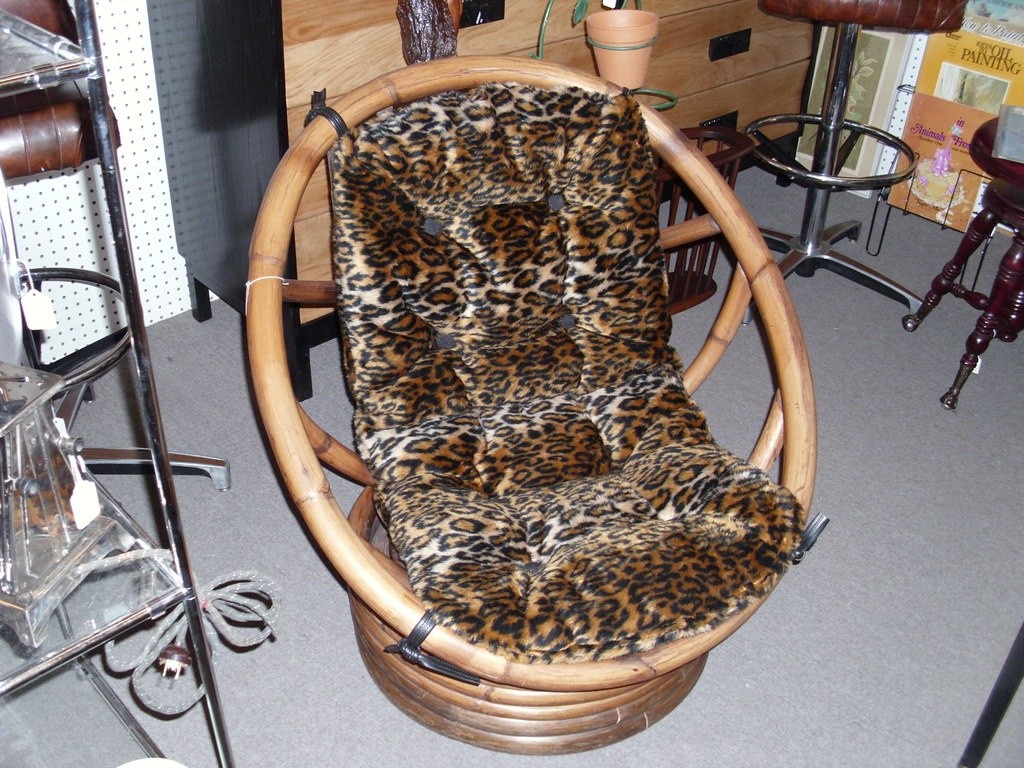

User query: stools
[0,0,229,639]
[739,0,971,325]
[902,117,1024,409]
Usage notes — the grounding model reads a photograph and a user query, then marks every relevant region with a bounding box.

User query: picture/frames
[795,26,915,199]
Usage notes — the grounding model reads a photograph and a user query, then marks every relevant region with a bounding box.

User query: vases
[587,10,658,91]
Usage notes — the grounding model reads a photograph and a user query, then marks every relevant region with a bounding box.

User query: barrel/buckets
[587,10,660,90]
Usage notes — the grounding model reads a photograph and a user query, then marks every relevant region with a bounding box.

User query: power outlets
[709,28,751,61]
[699,111,737,141]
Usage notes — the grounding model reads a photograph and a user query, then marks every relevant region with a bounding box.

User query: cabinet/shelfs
[0,0,233,768]
[148,0,822,400]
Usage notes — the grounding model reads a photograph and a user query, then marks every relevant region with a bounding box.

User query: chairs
[246,54,818,752]
